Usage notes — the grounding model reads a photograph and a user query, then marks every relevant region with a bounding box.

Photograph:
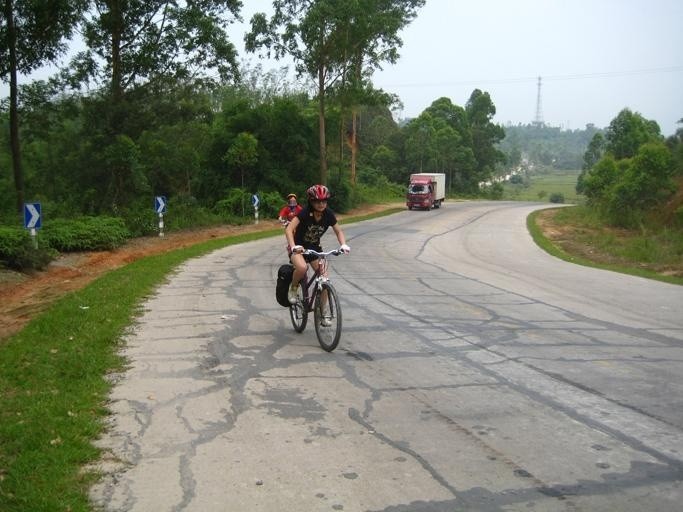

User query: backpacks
[275,264,296,308]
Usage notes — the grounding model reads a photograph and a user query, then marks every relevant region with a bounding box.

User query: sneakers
[287,282,300,305]
[321,310,333,327]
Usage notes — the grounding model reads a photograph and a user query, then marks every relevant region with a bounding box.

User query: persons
[283,184,351,327]
[277,193,301,245]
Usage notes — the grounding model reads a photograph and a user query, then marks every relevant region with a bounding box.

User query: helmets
[306,184,331,201]
[287,193,297,199]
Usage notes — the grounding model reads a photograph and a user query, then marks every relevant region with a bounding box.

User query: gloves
[339,243,351,252]
[281,219,289,226]
[290,245,302,254]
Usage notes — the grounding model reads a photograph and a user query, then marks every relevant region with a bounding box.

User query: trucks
[405,173,445,211]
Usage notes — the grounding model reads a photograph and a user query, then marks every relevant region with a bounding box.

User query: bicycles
[289,249,348,352]
